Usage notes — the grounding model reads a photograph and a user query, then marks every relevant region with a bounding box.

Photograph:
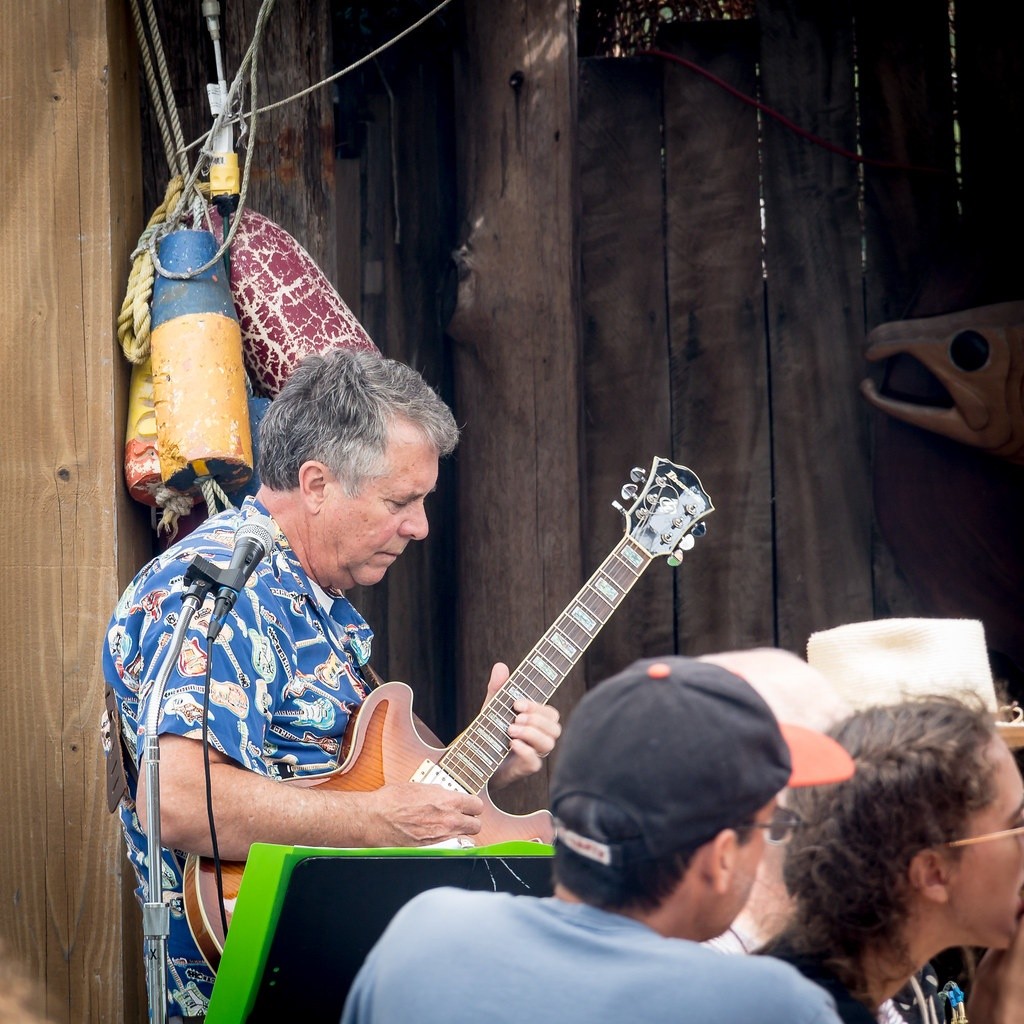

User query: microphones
[206,514,276,642]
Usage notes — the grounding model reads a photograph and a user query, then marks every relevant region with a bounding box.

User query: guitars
[182,453,717,982]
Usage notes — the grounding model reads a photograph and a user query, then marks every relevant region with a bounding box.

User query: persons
[753,689,1023,1022]
[341,655,844,1024]
[100,345,562,1023]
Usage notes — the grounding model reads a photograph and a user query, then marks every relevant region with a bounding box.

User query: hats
[548,657,854,867]
